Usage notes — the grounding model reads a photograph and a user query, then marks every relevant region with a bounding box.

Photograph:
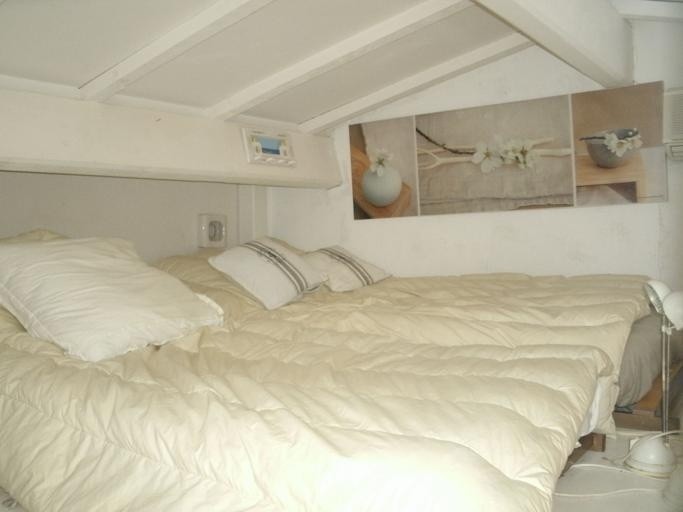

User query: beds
[151,255,683,414]
[0,228,617,511]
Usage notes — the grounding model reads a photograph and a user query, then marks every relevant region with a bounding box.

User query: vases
[362,164,402,208]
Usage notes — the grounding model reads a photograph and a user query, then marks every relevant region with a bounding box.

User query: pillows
[306,244,392,293]
[0,237,221,362]
[207,235,329,311]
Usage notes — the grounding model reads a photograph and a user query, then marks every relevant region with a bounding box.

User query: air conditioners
[662,87,683,161]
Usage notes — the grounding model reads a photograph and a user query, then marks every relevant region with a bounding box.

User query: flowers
[370,147,395,177]
[415,128,541,174]
[578,133,644,157]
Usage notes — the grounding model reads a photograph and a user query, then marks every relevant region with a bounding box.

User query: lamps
[624,280,683,474]
